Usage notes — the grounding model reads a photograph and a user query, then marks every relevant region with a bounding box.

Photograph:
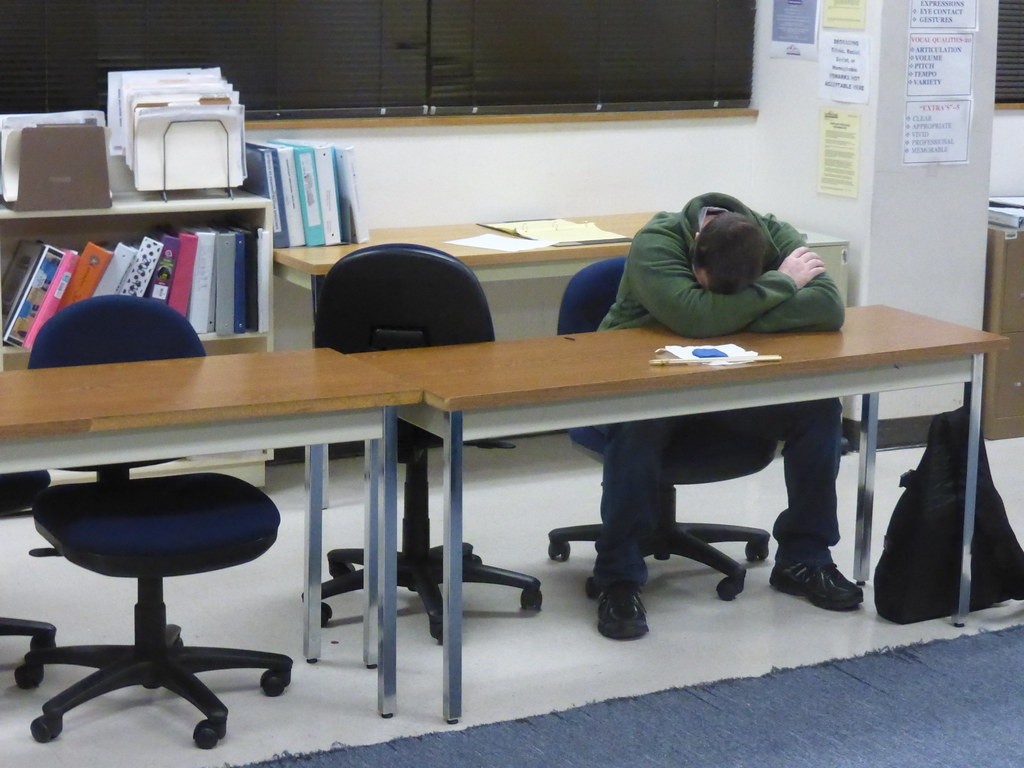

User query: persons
[589,192,862,640]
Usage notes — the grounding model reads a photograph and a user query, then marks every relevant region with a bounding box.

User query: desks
[795,230,850,422]
[0,347,425,717]
[345,306,1009,721]
[274,211,659,511]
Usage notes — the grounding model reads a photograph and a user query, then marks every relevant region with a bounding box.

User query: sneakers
[769,558,863,610]
[598,585,649,639]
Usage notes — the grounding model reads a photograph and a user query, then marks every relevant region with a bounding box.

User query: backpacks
[874,407,1024,624]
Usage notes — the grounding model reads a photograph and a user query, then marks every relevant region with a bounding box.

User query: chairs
[546,256,771,602]
[302,243,545,642]
[26,294,294,749]
[0,471,57,686]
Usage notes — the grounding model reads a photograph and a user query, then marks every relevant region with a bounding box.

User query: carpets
[228,623,1024,768]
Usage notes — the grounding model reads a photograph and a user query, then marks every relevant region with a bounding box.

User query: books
[989,206,1024,229]
[248,131,369,246]
[2,223,266,353]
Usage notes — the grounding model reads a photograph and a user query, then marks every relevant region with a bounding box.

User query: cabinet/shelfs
[0,191,274,489]
[981,225,1024,440]
